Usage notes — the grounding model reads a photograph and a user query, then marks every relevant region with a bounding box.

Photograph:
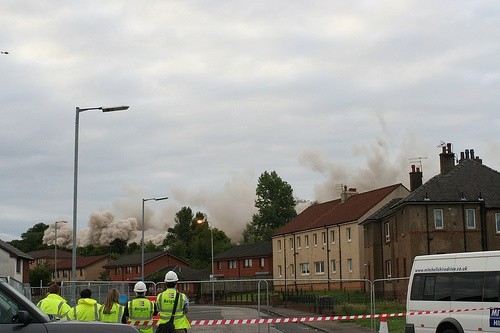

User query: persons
[122,281,159,333]
[98,288,125,323]
[156,271,191,333]
[36,284,71,320]
[65,288,102,321]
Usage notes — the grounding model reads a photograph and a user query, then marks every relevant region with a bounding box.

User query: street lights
[54,220,69,284]
[141,196,169,281]
[71,103,129,307]
[196,218,216,306]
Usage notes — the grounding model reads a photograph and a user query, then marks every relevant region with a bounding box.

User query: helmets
[133,281,147,292]
[164,271,179,282]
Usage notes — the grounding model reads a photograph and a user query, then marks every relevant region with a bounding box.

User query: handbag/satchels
[155,321,175,333]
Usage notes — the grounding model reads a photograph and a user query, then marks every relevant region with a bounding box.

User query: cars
[0,276,142,333]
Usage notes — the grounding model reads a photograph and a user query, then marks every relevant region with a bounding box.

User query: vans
[404,249,500,332]
